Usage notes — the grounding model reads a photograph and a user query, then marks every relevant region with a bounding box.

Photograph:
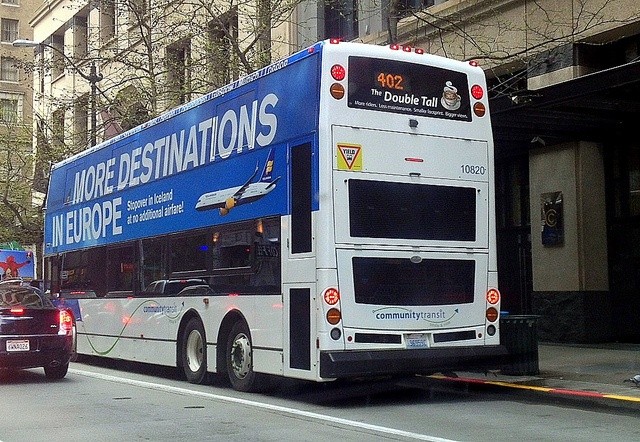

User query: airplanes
[194,147,282,216]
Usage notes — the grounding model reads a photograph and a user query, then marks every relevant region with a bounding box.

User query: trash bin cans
[499,313,542,374]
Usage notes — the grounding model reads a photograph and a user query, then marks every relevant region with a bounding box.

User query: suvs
[146,278,208,296]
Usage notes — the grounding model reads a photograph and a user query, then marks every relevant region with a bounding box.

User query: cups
[442,91,461,107]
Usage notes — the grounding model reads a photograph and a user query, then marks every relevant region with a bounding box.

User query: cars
[175,284,240,296]
[59,290,102,299]
[1,285,74,380]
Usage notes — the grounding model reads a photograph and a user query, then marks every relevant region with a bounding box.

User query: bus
[41,40,509,391]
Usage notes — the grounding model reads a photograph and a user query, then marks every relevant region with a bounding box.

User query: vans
[0,279,31,287]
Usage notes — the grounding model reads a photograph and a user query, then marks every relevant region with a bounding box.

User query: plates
[441,96,461,110]
